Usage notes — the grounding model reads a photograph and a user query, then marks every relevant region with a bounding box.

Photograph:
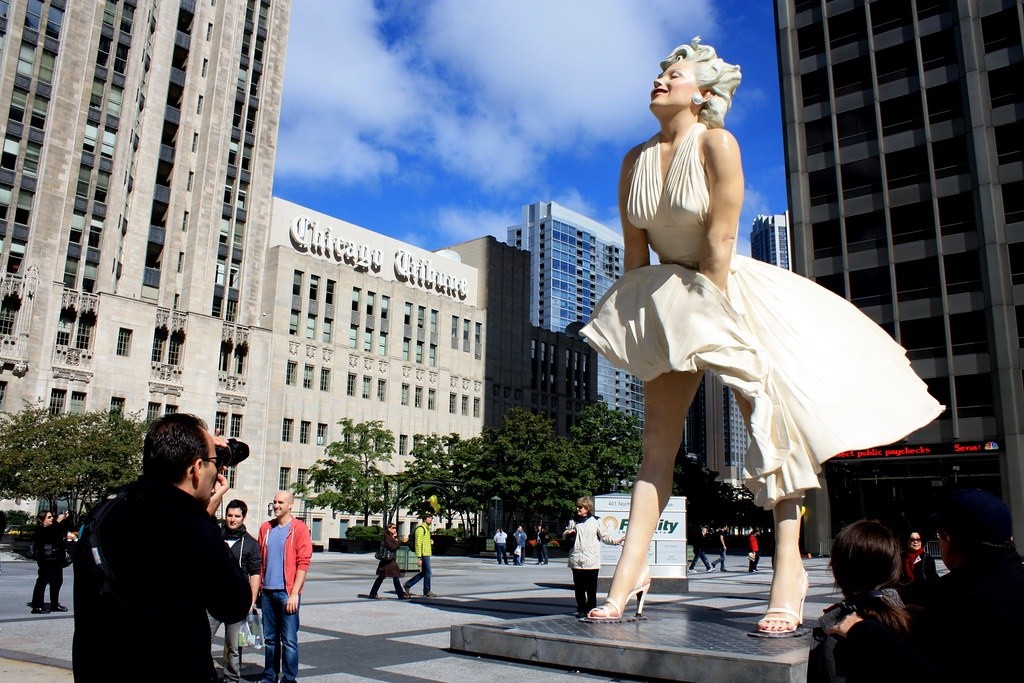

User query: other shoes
[750,568,757,573]
[707,567,714,572]
[399,595,411,599]
[424,590,436,597]
[51,603,67,612]
[712,562,715,568]
[721,569,726,571]
[687,569,697,573]
[403,583,411,596]
[256,678,274,683]
[369,593,381,600]
[32,606,50,614]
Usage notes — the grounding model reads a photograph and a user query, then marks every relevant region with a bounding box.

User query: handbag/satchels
[237,605,264,649]
[747,553,756,561]
[514,545,522,556]
[566,516,576,548]
[375,531,393,561]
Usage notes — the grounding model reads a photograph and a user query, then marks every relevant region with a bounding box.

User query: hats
[936,486,1021,568]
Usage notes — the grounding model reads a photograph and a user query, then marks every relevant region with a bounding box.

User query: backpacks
[407,525,425,551]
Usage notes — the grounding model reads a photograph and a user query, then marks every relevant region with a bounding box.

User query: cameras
[214,438,249,471]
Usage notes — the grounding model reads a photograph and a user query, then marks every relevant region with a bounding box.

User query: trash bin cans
[395,546,422,570]
[687,545,694,560]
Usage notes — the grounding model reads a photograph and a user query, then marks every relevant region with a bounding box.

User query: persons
[493,528,509,565]
[514,526,527,565]
[537,525,548,564]
[254,490,313,683]
[368,523,411,599]
[711,524,727,571]
[577,34,947,634]
[207,499,261,683]
[749,528,759,572]
[807,490,1024,683]
[563,496,625,618]
[31,510,74,614]
[403,514,435,596]
[687,527,715,572]
[72,413,253,683]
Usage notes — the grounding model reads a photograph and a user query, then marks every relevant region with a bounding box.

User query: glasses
[391,527,397,529]
[910,538,922,543]
[189,454,224,474]
[44,515,54,519]
[576,505,585,509]
